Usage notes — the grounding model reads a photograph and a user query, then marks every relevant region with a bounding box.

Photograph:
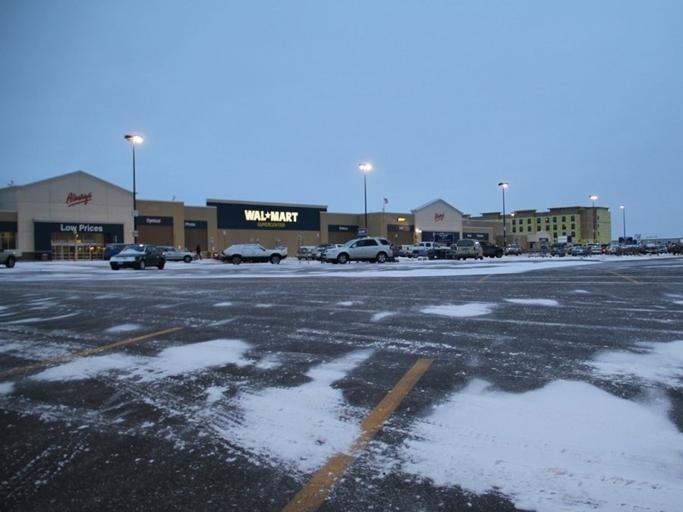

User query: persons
[196,244,202,260]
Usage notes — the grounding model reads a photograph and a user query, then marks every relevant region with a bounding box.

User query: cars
[296,237,521,264]
[213,243,287,265]
[0,243,16,268]
[550,241,683,256]
[102,243,197,270]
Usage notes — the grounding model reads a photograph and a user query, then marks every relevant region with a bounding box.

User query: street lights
[124,134,144,244]
[589,194,598,242]
[358,163,371,234]
[498,182,509,247]
[620,204,626,239]
